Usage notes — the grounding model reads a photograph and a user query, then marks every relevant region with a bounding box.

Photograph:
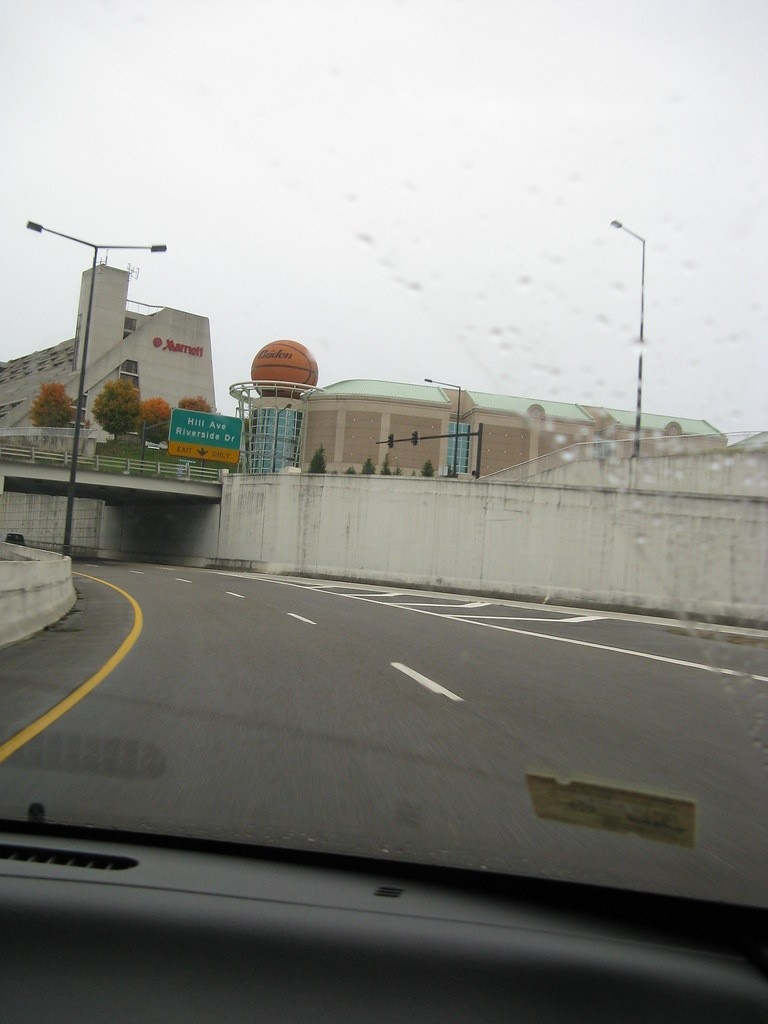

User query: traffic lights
[411,431,418,446]
[387,434,394,448]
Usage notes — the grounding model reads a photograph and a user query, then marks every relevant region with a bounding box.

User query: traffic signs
[168,408,244,465]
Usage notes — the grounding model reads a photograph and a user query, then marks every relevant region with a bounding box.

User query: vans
[0,532,25,546]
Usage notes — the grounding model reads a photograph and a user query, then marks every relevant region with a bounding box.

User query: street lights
[611,219,647,465]
[27,221,167,556]
[424,379,462,477]
